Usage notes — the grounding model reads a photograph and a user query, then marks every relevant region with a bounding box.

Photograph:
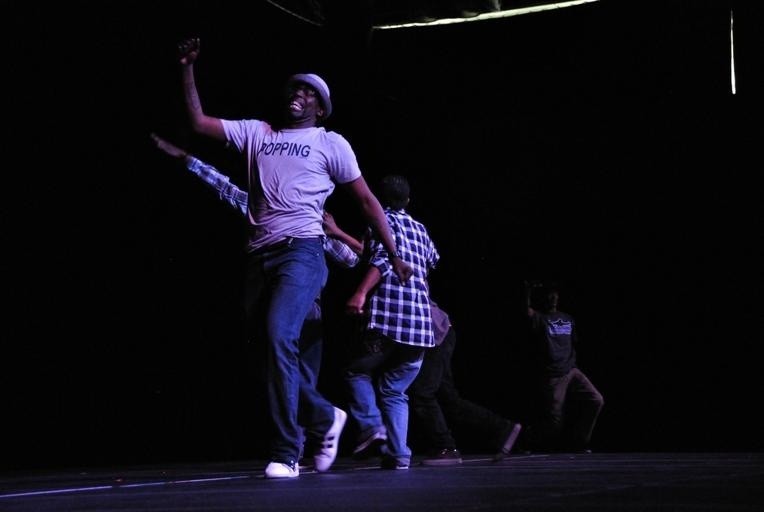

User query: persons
[528,283,604,454]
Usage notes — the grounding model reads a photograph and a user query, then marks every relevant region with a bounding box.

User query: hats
[289,74,332,119]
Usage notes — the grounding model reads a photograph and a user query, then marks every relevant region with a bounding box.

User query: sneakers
[265,462,299,478]
[496,421,521,460]
[354,432,462,469]
[314,406,347,472]
